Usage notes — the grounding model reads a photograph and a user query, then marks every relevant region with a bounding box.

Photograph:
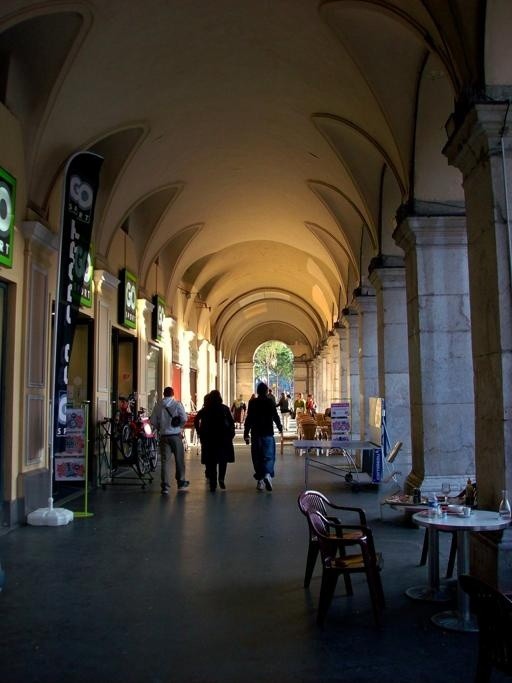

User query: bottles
[498,489,511,519]
[427,492,438,521]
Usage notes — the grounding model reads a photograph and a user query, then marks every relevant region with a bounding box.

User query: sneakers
[176,481,190,490]
[263,472,273,491]
[160,488,169,494]
[256,479,266,490]
[205,478,226,492]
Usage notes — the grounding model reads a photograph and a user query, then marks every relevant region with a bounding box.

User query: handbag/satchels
[170,415,182,428]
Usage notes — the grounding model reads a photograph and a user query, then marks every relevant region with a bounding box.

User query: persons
[286,393,292,405]
[191,388,236,492]
[267,387,276,401]
[149,386,190,494]
[294,391,306,433]
[249,392,256,404]
[229,392,247,430]
[275,391,292,431]
[306,393,319,415]
[201,392,212,479]
[242,381,283,492]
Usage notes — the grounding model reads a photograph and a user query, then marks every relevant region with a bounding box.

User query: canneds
[413,487,421,504]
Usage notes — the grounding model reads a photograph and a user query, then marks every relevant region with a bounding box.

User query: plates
[445,513,472,518]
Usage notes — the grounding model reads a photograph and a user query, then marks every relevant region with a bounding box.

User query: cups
[463,506,471,515]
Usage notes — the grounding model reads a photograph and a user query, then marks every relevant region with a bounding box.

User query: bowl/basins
[447,504,463,512]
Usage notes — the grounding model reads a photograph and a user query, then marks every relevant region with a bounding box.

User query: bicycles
[120,408,157,476]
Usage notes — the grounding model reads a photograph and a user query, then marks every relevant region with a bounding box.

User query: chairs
[306,507,385,611]
[280,428,300,456]
[297,489,371,589]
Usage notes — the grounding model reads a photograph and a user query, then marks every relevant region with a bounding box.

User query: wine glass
[440,481,451,505]
